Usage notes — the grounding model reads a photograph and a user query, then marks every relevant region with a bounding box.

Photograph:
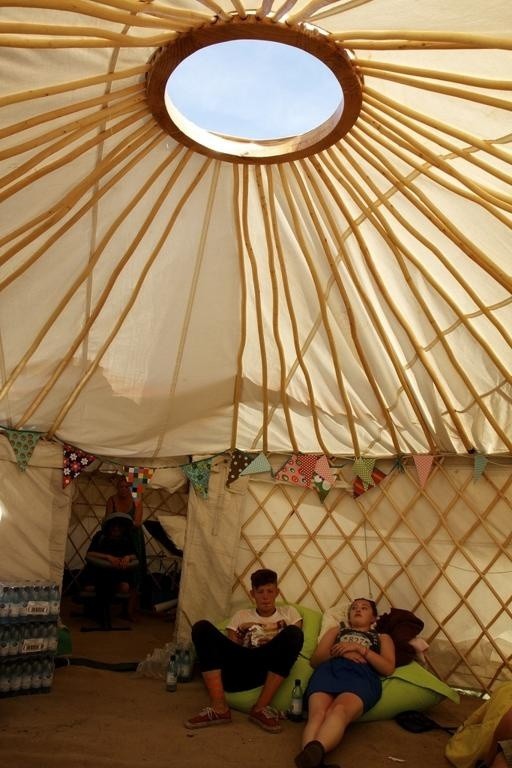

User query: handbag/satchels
[395,710,459,735]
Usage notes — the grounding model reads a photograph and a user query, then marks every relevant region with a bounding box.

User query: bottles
[0,579,62,696]
[165,655,176,692]
[172,648,181,676]
[289,678,304,723]
[181,651,191,684]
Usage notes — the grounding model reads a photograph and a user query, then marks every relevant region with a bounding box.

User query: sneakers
[294,739,326,767]
[184,705,232,728]
[248,703,282,733]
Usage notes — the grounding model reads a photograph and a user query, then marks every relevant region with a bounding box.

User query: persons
[182,567,306,733]
[295,596,399,767]
[104,474,144,621]
[84,516,140,627]
